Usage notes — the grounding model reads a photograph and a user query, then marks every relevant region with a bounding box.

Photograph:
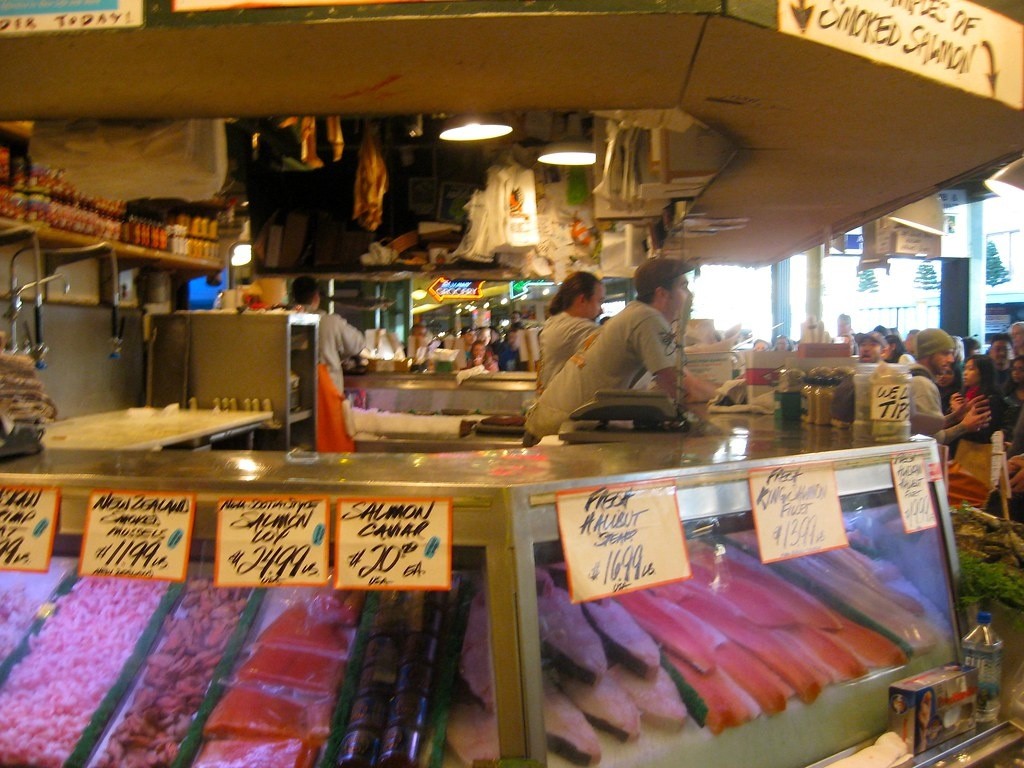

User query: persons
[944,355,1008,444]
[398,305,617,377]
[1003,452,1024,495]
[935,363,965,415]
[949,336,966,378]
[523,256,728,446]
[286,272,369,453]
[987,332,1015,442]
[538,270,609,411]
[1010,319,1024,358]
[746,314,920,370]
[907,328,994,445]
[1000,355,1024,444]
[963,337,986,361]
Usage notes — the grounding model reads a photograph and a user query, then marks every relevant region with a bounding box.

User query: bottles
[776,361,840,426]
[852,361,883,439]
[870,361,912,442]
[960,611,1003,722]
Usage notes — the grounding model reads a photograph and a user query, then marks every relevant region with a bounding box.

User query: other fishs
[447,511,940,768]
[187,586,364,768]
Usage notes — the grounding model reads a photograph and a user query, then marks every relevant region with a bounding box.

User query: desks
[42,410,273,447]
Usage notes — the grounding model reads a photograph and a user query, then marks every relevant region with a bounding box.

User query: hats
[635,258,696,297]
[913,329,955,359]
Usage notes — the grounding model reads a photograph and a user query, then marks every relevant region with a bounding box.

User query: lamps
[439,113,512,142]
[539,139,598,166]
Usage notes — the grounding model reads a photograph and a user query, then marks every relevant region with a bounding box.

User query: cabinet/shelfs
[153,307,319,449]
[0,217,221,308]
[264,272,410,375]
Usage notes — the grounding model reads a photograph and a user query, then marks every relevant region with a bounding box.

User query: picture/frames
[438,182,470,222]
[409,177,439,214]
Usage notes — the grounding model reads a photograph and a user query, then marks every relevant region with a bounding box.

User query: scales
[569,388,679,433]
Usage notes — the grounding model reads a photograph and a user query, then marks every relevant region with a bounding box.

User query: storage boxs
[799,342,851,376]
[745,348,792,406]
[682,350,732,403]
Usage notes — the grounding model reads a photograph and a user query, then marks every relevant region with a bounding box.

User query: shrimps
[0,575,250,767]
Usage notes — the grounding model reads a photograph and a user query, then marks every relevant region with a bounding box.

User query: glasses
[991,346,1011,351]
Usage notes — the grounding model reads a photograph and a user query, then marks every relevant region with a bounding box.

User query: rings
[978,427,981,432]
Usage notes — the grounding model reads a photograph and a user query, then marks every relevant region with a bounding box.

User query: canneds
[800,377,835,426]
[0,149,220,263]
[340,592,445,767]
